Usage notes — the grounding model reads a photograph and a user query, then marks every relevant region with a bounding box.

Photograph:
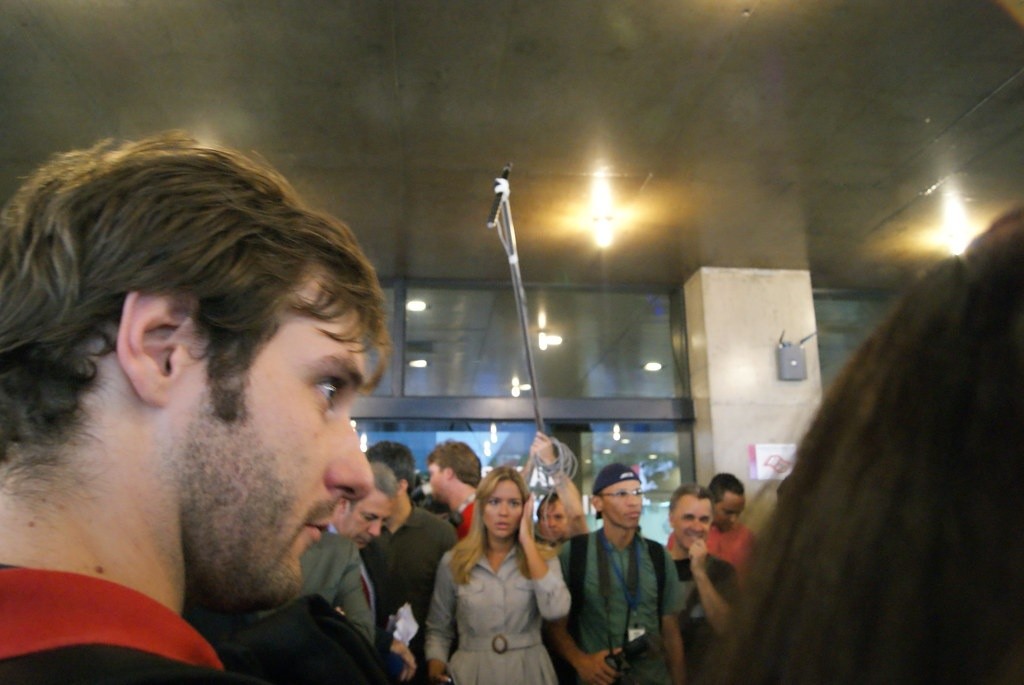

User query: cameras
[605,632,657,674]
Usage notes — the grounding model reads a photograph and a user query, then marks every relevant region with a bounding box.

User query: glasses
[595,488,643,500]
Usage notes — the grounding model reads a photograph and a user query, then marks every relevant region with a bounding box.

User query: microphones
[488,167,511,227]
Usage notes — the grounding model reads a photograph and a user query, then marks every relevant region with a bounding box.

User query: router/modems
[777,331,816,381]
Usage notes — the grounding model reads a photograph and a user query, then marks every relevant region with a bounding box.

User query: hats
[592,464,642,495]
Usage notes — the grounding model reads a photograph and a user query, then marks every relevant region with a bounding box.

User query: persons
[0,135,389,685]
[183,431,782,685]
[688,205,1024,685]
